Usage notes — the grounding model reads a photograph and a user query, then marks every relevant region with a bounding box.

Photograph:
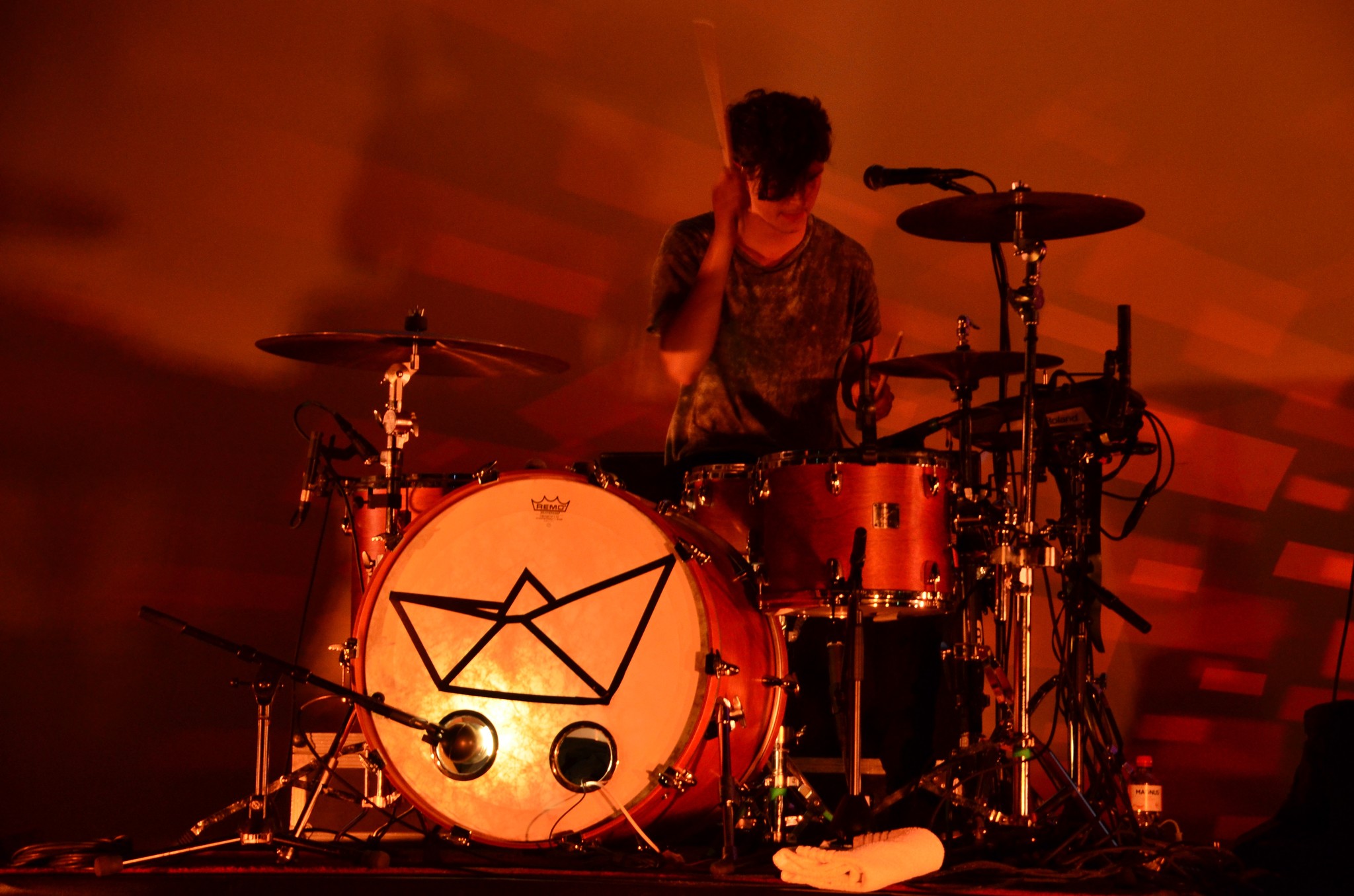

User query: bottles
[1127,756,1165,871]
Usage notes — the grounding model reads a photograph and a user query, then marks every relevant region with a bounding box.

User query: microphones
[437,724,479,764]
[862,163,975,192]
[330,408,379,466]
[297,427,323,515]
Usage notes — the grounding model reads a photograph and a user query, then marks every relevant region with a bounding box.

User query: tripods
[114,607,440,860]
[861,251,1130,860]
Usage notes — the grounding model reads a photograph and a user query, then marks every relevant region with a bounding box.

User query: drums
[743,448,980,618]
[281,473,481,723]
[680,447,806,579]
[344,467,788,845]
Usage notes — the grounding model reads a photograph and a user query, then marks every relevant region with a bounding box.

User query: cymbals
[253,331,572,380]
[865,351,1065,383]
[895,190,1146,244]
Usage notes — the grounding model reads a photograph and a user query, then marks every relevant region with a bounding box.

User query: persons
[651,86,894,820]
